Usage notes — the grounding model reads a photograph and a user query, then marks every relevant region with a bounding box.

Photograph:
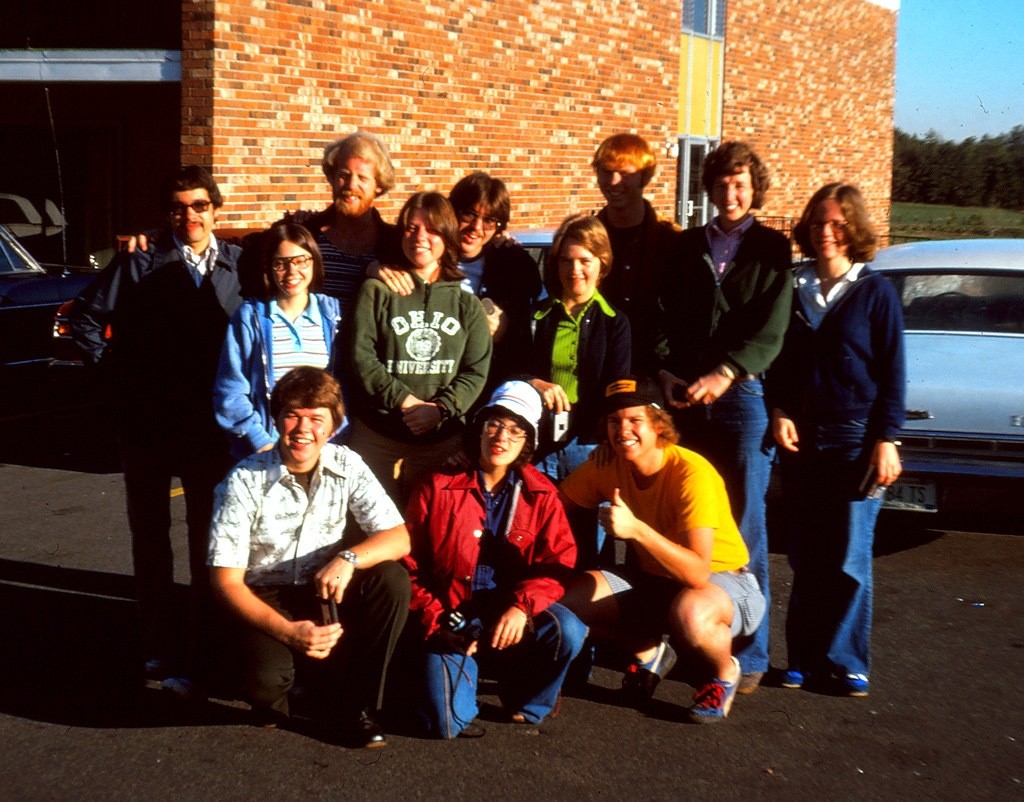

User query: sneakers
[690,655,742,725]
[623,639,677,701]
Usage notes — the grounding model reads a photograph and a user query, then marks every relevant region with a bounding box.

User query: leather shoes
[339,696,387,748]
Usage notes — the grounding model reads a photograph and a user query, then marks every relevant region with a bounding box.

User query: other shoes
[144,648,182,691]
[736,670,763,695]
[783,668,806,688]
[847,672,869,697]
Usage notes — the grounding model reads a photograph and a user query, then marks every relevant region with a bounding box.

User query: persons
[764,183,905,696]
[212,222,351,455]
[119,134,520,337]
[527,375,768,722]
[520,214,632,553]
[637,140,793,694]
[201,365,412,748]
[371,172,543,381]
[72,165,245,691]
[399,380,588,739]
[339,191,493,552]
[589,132,686,374]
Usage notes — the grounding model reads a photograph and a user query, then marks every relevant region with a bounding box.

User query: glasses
[484,419,528,442]
[271,255,314,272]
[807,218,849,237]
[168,200,211,214]
[457,206,502,230]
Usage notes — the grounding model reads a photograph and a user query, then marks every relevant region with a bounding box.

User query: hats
[486,381,542,449]
[606,380,664,410]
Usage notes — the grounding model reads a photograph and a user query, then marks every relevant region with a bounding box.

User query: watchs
[336,550,359,568]
[431,398,450,431]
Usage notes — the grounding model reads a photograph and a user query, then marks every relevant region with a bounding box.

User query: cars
[0,223,104,425]
[859,237,1024,524]
[498,232,559,302]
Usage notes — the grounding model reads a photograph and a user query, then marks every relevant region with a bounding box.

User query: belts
[728,372,760,388]
